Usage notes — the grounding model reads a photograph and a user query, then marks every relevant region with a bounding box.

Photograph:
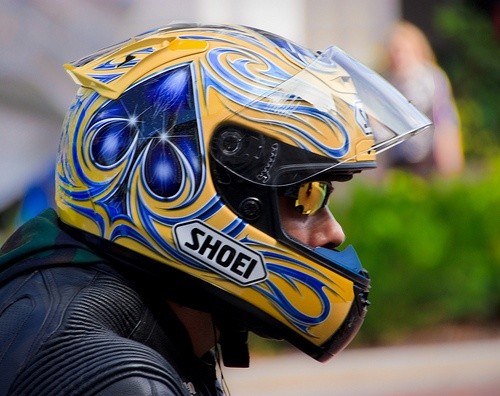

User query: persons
[0,18,436,396]
[373,21,463,179]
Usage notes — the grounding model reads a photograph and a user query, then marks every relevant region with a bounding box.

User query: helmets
[53,20,434,364]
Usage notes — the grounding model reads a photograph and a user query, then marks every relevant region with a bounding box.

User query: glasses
[272,176,336,217]
